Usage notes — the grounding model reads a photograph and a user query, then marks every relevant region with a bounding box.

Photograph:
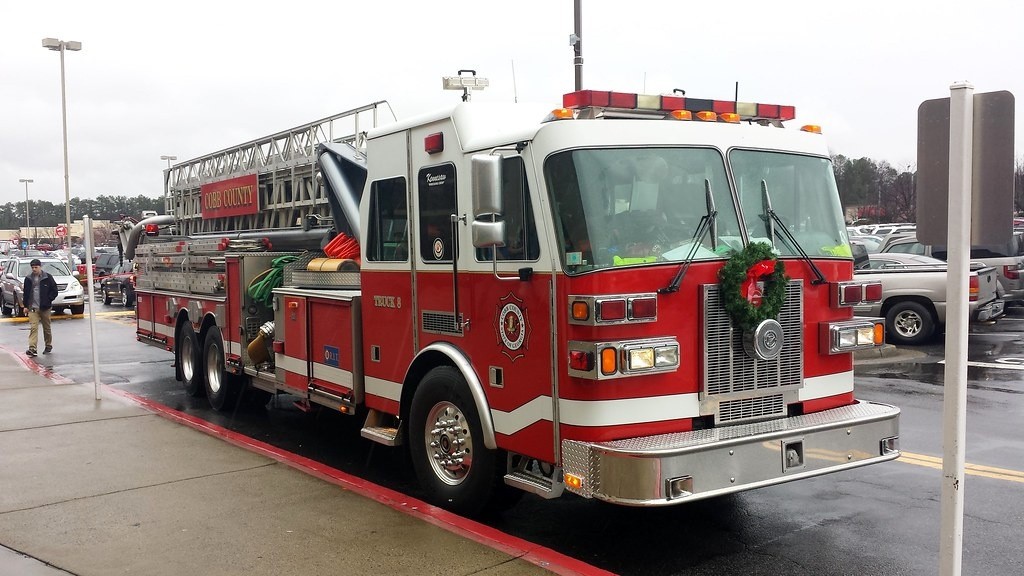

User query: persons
[22,259,58,356]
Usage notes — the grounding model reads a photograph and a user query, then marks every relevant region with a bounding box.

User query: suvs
[0,257,85,318]
[872,232,1024,317]
[95,254,122,282]
[100,261,137,306]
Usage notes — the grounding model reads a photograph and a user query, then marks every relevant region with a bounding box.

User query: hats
[30,259,41,267]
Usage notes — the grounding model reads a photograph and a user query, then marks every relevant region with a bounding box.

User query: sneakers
[43,345,53,355]
[26,349,38,358]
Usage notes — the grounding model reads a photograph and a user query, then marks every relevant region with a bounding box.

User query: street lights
[43,37,81,271]
[19,179,35,247]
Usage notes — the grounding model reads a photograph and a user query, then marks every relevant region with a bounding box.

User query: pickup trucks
[36,244,53,251]
[849,241,1006,344]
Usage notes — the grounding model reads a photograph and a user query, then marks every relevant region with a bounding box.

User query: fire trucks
[114,66,900,516]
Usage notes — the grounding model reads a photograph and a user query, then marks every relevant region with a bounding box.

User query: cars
[845,223,916,253]
[0,242,117,275]
[1014,216,1024,233]
